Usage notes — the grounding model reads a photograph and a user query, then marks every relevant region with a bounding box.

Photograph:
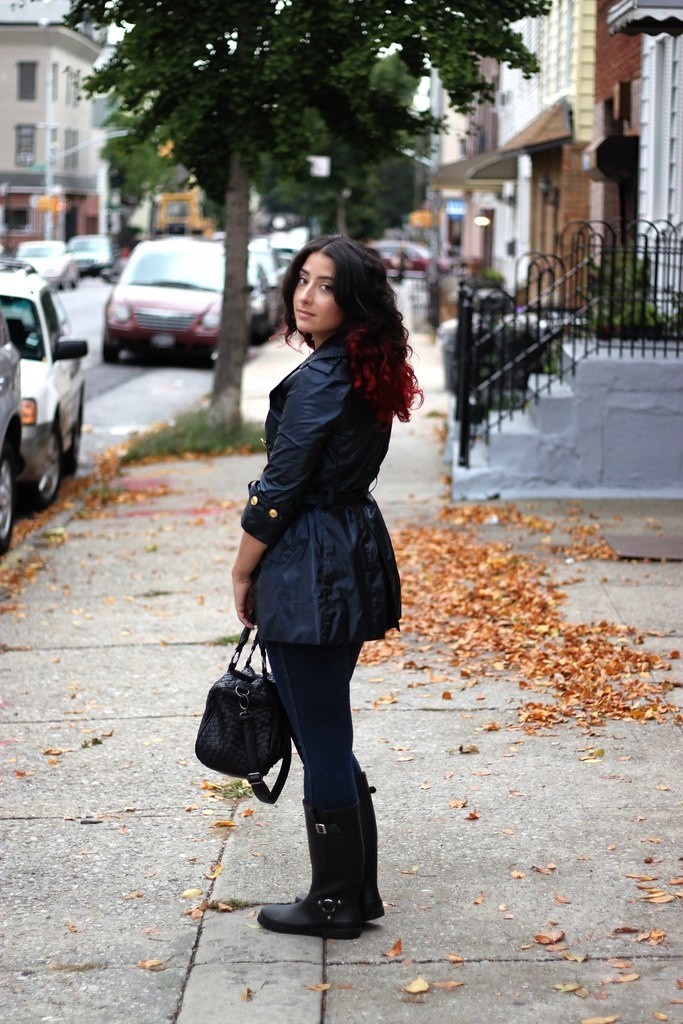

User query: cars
[234,256,274,350]
[368,240,455,279]
[103,236,256,368]
[266,223,310,273]
[67,233,129,284]
[241,237,292,336]
[13,239,79,290]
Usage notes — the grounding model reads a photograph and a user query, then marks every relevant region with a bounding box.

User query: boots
[256,770,385,940]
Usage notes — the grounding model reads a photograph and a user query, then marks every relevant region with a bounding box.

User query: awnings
[429,98,575,193]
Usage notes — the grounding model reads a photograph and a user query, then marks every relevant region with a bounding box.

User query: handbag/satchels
[194,604,291,805]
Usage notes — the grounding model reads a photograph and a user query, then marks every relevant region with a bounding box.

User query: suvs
[0,256,89,506]
[0,309,26,554]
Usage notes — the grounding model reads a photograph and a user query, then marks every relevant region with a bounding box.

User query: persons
[231,234,416,937]
